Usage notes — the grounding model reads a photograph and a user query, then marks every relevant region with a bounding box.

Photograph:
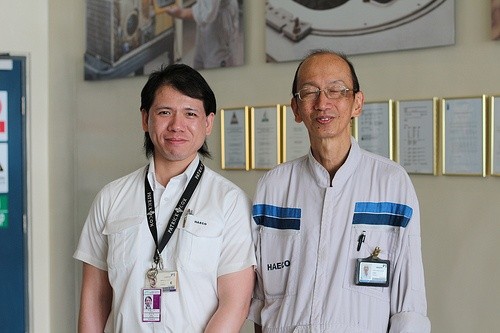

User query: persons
[72,62,257,333]
[165,1,242,67]
[250,47,432,332]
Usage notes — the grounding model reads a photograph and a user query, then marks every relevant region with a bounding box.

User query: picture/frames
[487,94,500,178]
[251,103,282,171]
[354,98,394,164]
[394,96,439,177]
[218,105,251,173]
[439,93,488,179]
[283,103,311,163]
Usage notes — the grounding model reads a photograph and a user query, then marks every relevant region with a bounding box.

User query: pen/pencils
[182,209,192,227]
[357,230,367,252]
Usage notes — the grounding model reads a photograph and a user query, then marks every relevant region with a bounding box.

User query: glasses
[295,84,357,102]
